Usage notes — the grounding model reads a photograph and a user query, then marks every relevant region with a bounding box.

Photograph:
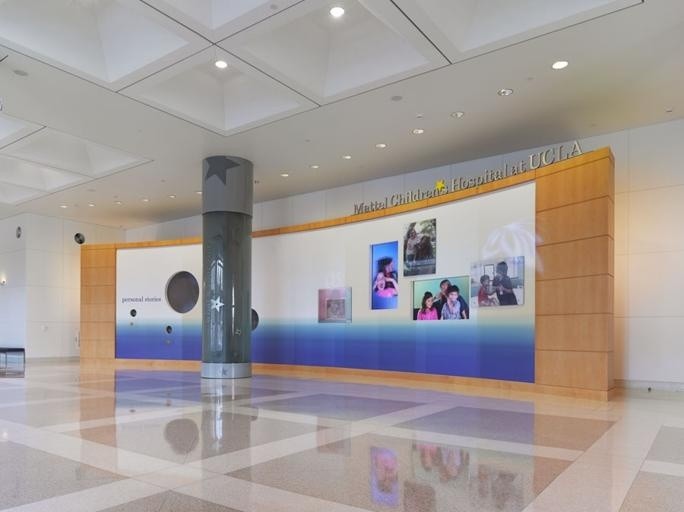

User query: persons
[431,279,469,320]
[490,262,518,304]
[375,256,397,289]
[417,291,438,320]
[416,227,433,260]
[439,284,467,321]
[407,230,420,267]
[369,444,519,511]
[370,272,398,298]
[478,275,500,306]
